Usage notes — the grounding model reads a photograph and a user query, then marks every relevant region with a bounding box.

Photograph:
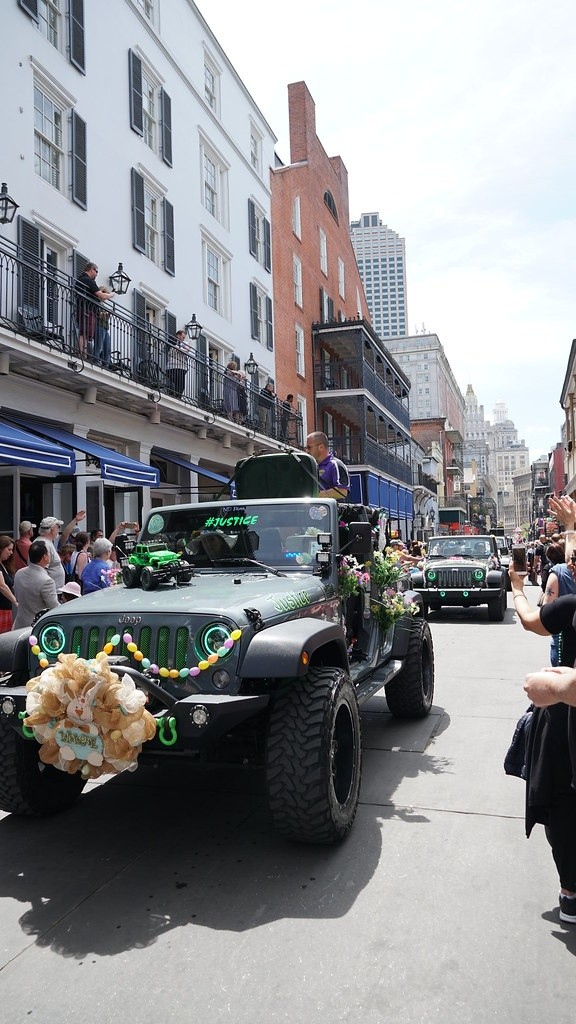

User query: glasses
[92,268,99,274]
[306,443,323,450]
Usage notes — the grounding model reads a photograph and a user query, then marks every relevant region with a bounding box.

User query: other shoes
[559,894,576,923]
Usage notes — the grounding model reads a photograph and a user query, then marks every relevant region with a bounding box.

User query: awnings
[0,411,160,486]
[0,420,76,473]
[151,451,235,499]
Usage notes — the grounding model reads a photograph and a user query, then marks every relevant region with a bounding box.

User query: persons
[223,361,248,426]
[306,431,350,498]
[259,383,277,438]
[75,261,111,368]
[281,394,295,440]
[386,494,576,923]
[0,510,140,633]
[166,330,188,399]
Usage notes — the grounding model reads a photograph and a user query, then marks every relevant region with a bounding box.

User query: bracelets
[135,529,140,532]
[513,595,527,600]
[560,530,575,538]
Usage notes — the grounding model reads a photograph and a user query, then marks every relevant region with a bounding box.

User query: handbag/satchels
[506,711,536,780]
[99,315,110,329]
[63,573,80,586]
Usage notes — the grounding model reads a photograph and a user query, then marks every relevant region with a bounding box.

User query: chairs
[322,375,340,390]
[18,307,65,350]
[201,392,224,415]
[101,351,132,378]
[139,360,172,395]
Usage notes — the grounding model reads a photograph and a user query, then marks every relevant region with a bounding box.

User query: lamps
[222,434,231,448]
[246,443,254,455]
[428,507,435,523]
[244,353,258,374]
[109,263,131,294]
[185,314,202,340]
[0,351,10,376]
[150,410,160,423]
[197,428,206,439]
[0,183,20,224]
[83,387,97,404]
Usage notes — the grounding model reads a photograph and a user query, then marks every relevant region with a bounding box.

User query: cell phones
[511,545,527,576]
[125,523,136,529]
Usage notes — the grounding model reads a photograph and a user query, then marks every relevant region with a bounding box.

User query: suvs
[0,451,436,850]
[493,536,513,591]
[411,535,507,622]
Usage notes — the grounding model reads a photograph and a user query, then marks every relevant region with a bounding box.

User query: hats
[40,516,63,528]
[286,527,325,551]
[59,582,82,597]
[18,521,36,533]
[70,525,80,537]
[390,538,404,546]
